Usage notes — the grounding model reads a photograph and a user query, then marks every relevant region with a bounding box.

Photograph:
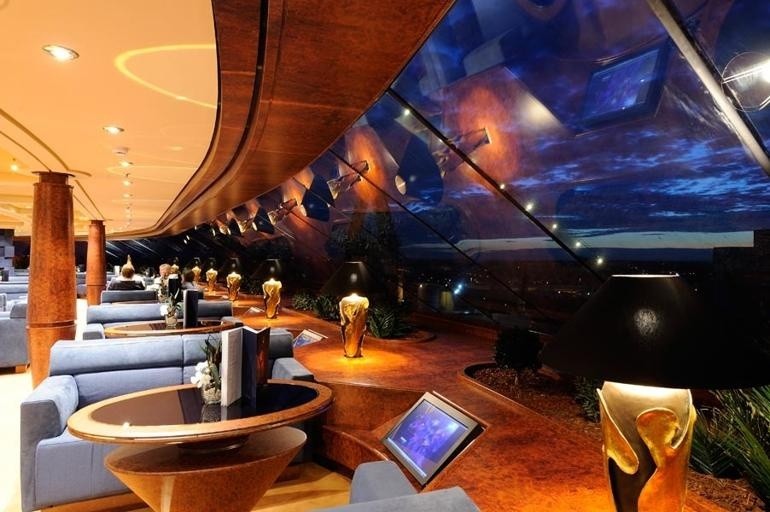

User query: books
[218,325,272,408]
[166,278,182,303]
[181,288,200,327]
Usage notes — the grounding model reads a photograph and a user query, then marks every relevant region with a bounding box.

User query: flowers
[189,350,221,407]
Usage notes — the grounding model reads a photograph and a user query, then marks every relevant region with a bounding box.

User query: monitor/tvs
[380,390,480,487]
[244,307,261,315]
[575,33,674,136]
[292,329,323,349]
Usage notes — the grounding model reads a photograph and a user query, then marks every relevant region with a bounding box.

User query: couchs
[20,327,315,510]
[83,301,234,340]
[101,264,204,301]
[317,459,482,512]
[0,266,87,375]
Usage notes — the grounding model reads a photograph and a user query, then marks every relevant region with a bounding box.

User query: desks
[105,319,231,339]
[65,380,332,509]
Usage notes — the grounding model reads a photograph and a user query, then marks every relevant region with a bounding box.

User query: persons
[181,271,200,289]
[106,263,146,290]
[155,263,178,286]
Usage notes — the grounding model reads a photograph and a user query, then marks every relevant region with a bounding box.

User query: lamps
[300,174,357,223]
[268,201,294,226]
[395,170,421,196]
[251,207,275,235]
[227,218,247,237]
